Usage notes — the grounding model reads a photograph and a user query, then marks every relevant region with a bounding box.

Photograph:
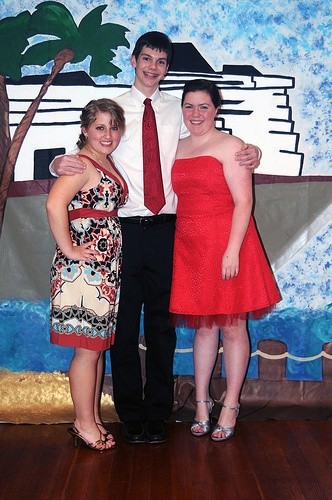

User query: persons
[46,97,129,452]
[46,31,262,445]
[171,78,284,442]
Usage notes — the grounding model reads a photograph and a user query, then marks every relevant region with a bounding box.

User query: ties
[142,98,166,215]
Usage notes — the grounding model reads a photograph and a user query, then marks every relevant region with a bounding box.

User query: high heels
[66,419,112,451]
[191,396,215,436]
[96,422,117,445]
[211,402,241,441]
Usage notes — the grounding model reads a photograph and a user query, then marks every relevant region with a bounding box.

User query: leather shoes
[122,421,146,444]
[145,420,168,444]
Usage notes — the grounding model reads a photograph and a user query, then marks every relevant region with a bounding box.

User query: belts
[118,214,176,227]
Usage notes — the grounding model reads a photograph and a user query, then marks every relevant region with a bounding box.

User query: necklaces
[84,146,110,171]
